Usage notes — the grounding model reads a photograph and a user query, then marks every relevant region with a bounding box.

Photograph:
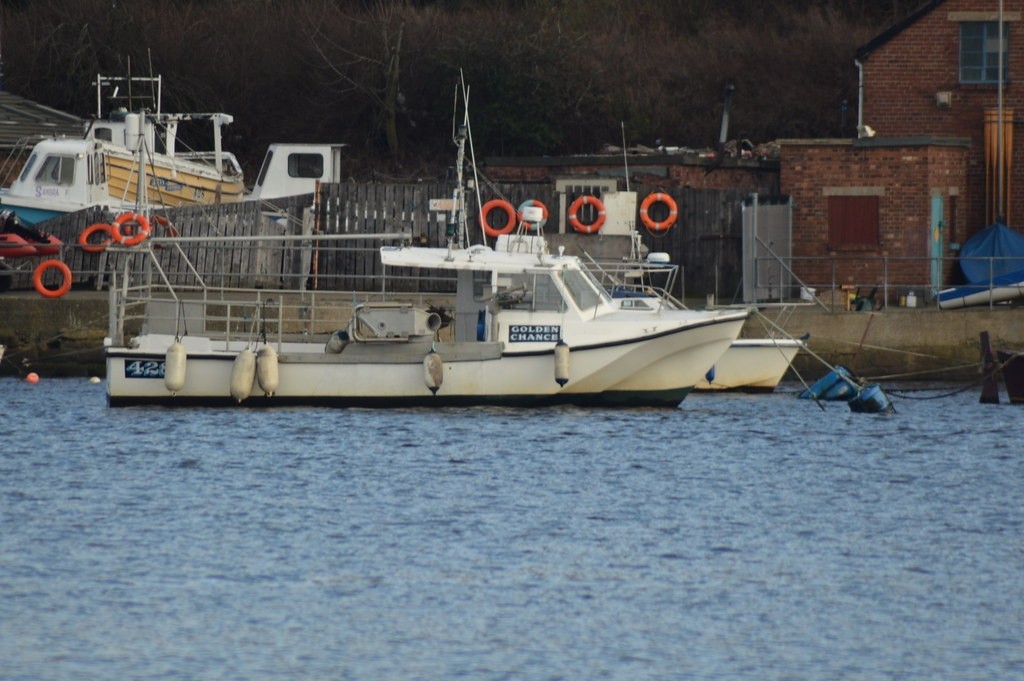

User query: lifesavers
[80,222,114,252]
[516,198,549,233]
[112,215,149,245]
[569,193,606,235]
[32,259,73,298]
[124,212,178,251]
[638,190,679,231]
[476,200,516,239]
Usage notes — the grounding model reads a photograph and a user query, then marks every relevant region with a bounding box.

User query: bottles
[907,292,917,307]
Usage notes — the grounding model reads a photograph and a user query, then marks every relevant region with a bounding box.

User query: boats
[936,268,1024,314]
[308,194,461,239]
[0,73,358,234]
[994,349,1024,405]
[103,68,754,407]
[580,259,811,393]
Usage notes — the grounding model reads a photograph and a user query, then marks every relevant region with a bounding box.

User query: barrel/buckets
[800,367,855,401]
[801,287,816,302]
[848,386,895,414]
